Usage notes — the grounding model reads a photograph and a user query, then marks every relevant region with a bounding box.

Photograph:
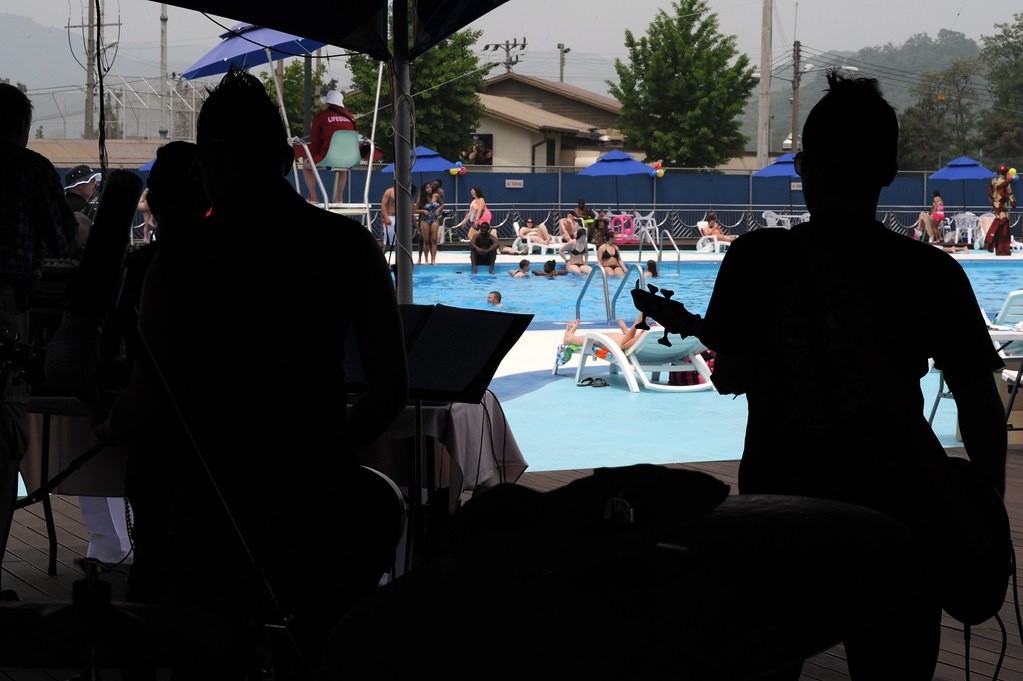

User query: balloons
[648,161,664,178]
[1009,168,1019,181]
[449,162,467,175]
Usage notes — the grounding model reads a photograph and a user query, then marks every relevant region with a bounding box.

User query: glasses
[527,221,533,223]
[790,151,888,183]
[425,185,432,189]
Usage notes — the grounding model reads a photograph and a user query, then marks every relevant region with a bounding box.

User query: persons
[301,89,357,203]
[0,62,410,681]
[462,187,530,275]
[487,291,502,307]
[929,189,969,254]
[532,260,568,277]
[643,260,659,278]
[508,259,530,278]
[409,179,445,265]
[562,312,663,351]
[705,213,738,244]
[557,199,614,250]
[598,231,628,278]
[137,188,156,244]
[380,178,397,254]
[559,228,593,274]
[690,69,1009,681]
[517,216,553,245]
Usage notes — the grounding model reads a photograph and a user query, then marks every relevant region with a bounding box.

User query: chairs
[800,212,810,223]
[695,221,731,253]
[437,216,455,245]
[314,130,362,203]
[762,210,791,227]
[551,326,718,392]
[513,209,660,255]
[921,211,996,244]
[928,289,1023,443]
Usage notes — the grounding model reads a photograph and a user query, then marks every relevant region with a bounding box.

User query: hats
[62,165,102,191]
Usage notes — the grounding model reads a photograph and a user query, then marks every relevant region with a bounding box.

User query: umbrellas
[927,156,998,214]
[139,158,157,172]
[752,153,802,215]
[380,146,459,182]
[576,148,657,214]
[178,23,330,195]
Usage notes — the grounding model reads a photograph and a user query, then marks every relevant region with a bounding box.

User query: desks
[14,389,530,579]
[777,214,801,218]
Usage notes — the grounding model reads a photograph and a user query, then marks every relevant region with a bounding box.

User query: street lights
[751,65,861,156]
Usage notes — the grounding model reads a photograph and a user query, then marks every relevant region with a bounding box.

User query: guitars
[630,279,1011,625]
